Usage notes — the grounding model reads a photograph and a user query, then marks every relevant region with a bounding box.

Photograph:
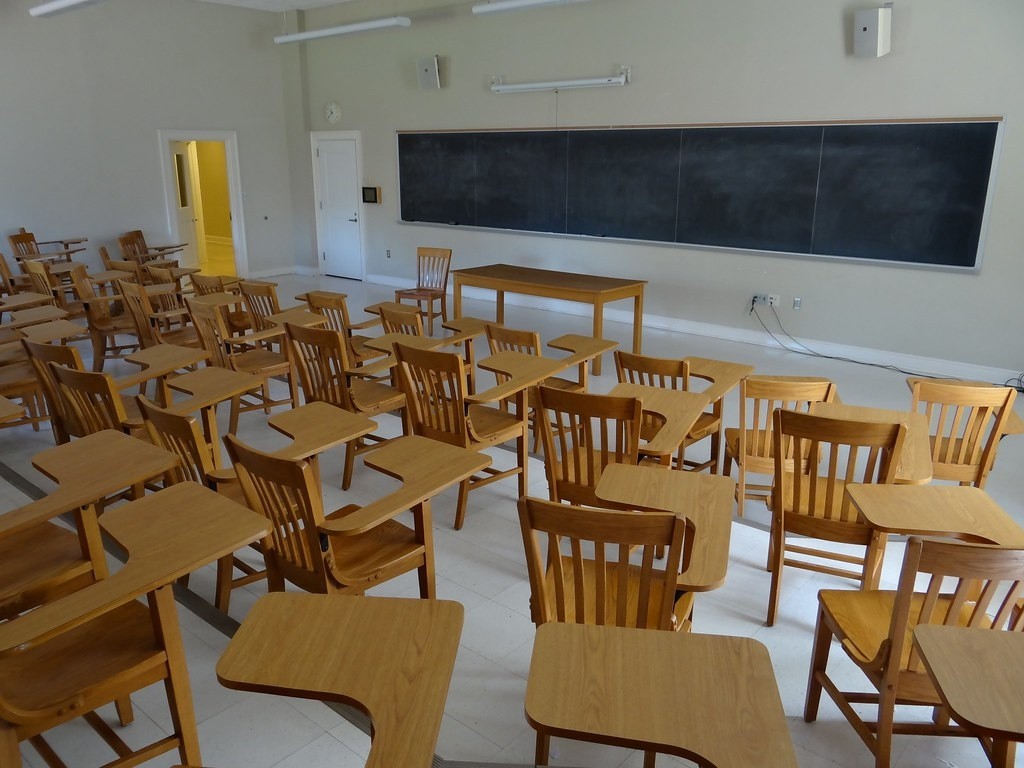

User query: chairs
[0,229,1023,768]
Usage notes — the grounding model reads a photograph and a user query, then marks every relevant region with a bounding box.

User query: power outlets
[751,294,767,307]
[767,295,779,308]
[387,250,390,258]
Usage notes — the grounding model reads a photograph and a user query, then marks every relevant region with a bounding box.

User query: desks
[449,263,649,376]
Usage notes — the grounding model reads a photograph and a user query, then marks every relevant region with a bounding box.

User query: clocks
[323,101,341,124]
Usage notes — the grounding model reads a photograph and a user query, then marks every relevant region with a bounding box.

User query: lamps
[273,16,411,44]
[472,0,565,17]
[30,0,95,19]
[491,66,631,93]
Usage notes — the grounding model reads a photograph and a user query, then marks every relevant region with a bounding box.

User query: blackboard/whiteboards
[395,116,999,276]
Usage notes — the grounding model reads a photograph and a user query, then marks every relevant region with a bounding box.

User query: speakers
[853,7,891,59]
[417,55,440,89]
